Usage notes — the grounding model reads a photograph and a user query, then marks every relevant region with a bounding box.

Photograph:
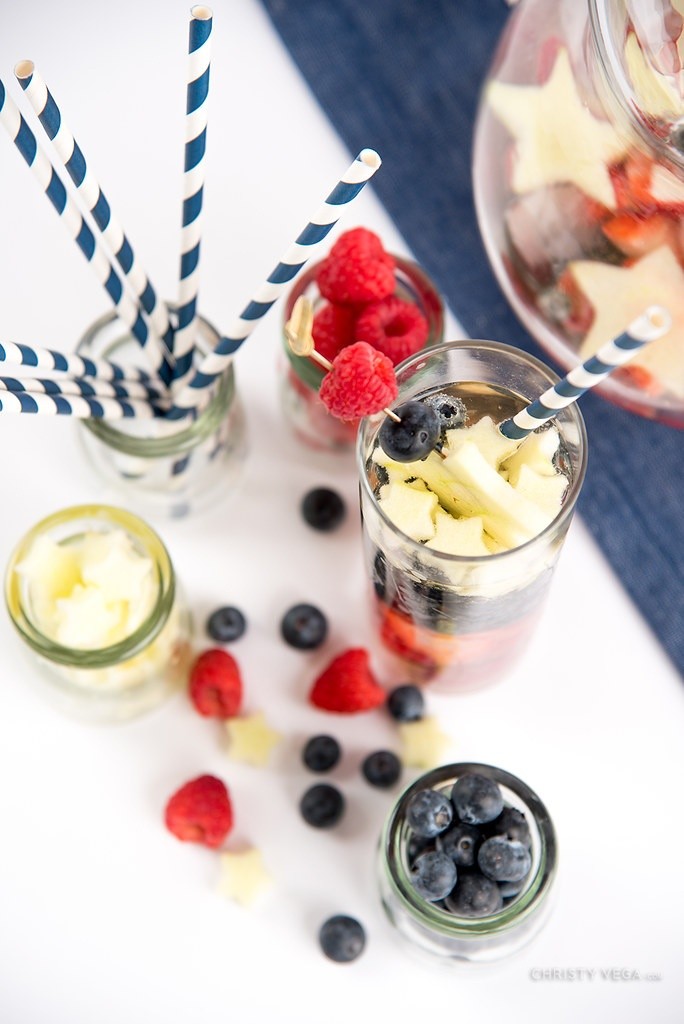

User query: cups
[377,762,563,958]
[278,252,446,457]
[468,0,684,434]
[66,303,238,490]
[2,502,197,711]
[355,338,587,669]
